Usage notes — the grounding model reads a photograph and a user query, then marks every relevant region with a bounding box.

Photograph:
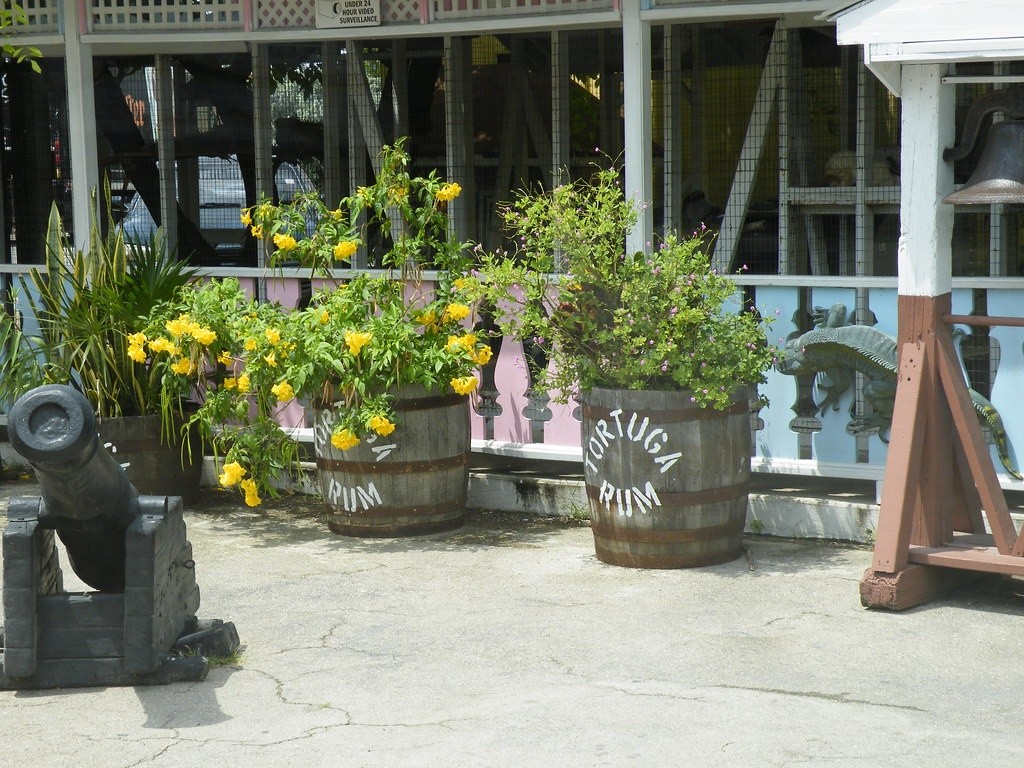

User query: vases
[312,375,471,537]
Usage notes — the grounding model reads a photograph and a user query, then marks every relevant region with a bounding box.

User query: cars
[112,156,332,269]
[110,169,138,209]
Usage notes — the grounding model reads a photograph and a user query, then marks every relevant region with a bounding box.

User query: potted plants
[450,145,806,569]
[0,224,215,506]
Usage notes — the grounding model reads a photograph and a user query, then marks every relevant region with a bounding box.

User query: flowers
[125,137,494,507]
